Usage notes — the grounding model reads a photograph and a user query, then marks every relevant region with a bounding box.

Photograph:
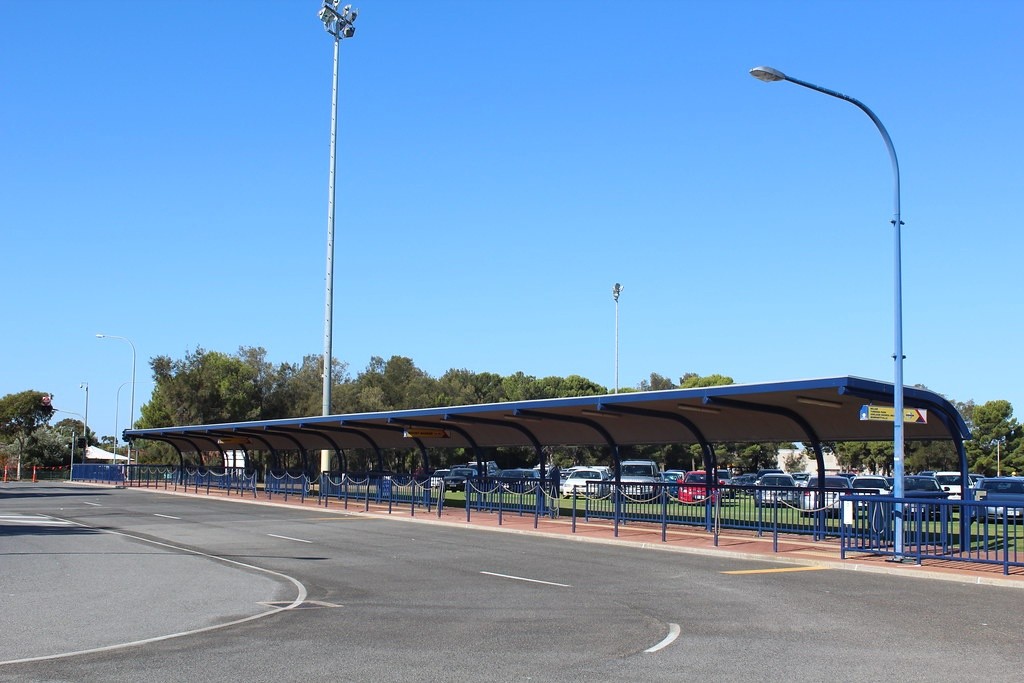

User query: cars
[443,467,480,493]
[850,476,893,509]
[561,470,612,501]
[934,472,977,511]
[430,465,994,505]
[883,476,951,522]
[972,476,1024,521]
[798,476,854,518]
[753,473,799,506]
[677,471,725,506]
[609,461,666,504]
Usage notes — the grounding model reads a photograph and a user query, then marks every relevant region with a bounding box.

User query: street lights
[113,381,157,464]
[96,333,136,481]
[749,65,907,565]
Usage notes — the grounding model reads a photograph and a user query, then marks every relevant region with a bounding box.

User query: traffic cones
[32,466,38,483]
[3,465,10,483]
